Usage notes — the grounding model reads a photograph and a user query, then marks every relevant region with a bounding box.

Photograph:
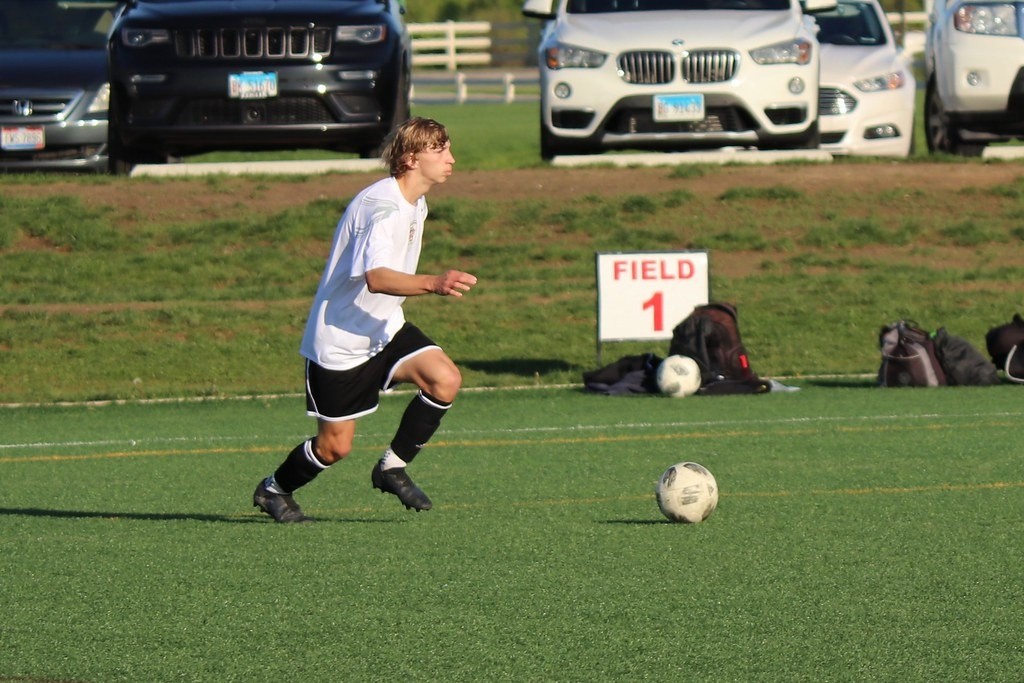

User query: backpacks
[931,324,999,388]
[583,353,665,396]
[669,303,772,396]
[876,319,947,386]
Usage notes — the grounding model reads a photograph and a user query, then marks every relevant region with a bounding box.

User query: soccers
[655,353,702,397]
[654,461,720,524]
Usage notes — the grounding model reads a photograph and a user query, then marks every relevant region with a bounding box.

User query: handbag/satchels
[985,312,1024,385]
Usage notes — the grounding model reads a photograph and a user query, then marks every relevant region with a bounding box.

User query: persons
[252,118,478,524]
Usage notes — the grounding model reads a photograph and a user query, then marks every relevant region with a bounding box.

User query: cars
[519,0,839,164]
[0,0,112,160]
[924,1,1024,162]
[805,1,921,164]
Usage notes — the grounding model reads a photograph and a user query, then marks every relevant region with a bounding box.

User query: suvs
[103,1,416,162]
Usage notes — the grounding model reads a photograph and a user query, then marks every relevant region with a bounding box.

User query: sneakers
[371,459,432,512]
[253,477,304,524]
